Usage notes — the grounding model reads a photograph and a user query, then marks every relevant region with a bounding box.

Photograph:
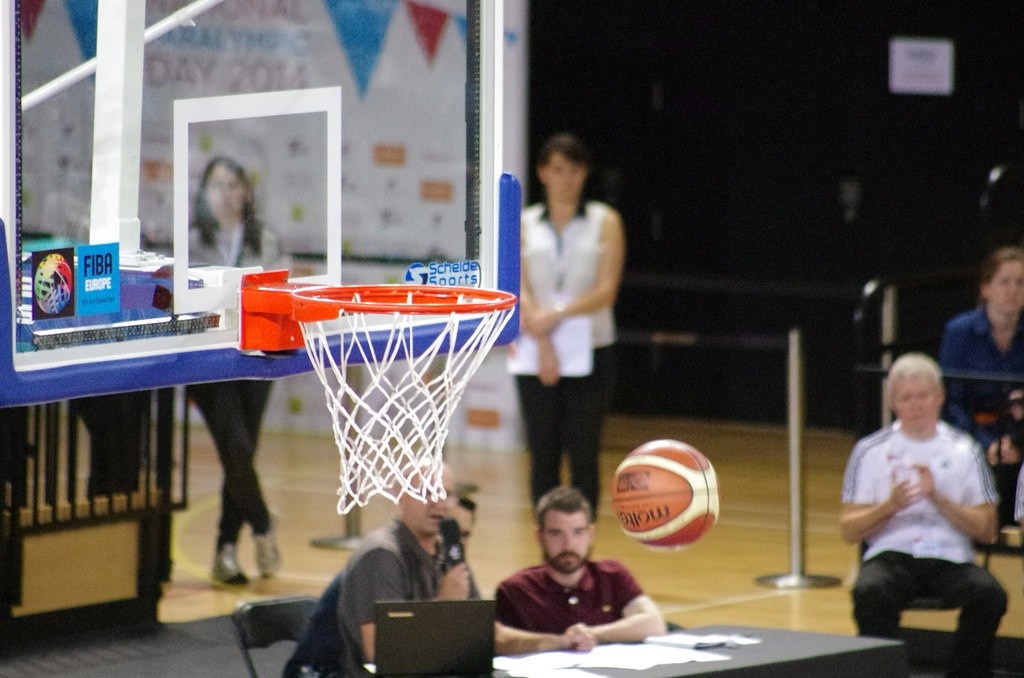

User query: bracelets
[553,301,568,312]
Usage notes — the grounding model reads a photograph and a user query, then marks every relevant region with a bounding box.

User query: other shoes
[214,547,246,582]
[254,514,280,577]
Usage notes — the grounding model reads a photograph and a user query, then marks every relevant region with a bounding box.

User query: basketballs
[610,439,722,549]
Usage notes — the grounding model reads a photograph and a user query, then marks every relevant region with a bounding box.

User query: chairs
[229,596,316,678]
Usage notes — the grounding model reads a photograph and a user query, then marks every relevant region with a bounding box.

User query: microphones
[441,518,463,568]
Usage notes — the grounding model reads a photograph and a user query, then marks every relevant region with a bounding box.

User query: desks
[488,628,913,678]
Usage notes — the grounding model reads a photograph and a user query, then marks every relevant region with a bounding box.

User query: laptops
[363,598,497,678]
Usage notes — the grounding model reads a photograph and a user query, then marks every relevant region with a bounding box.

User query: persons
[938,246,1023,552]
[838,352,1011,678]
[189,154,281,583]
[280,459,666,678]
[507,134,625,524]
